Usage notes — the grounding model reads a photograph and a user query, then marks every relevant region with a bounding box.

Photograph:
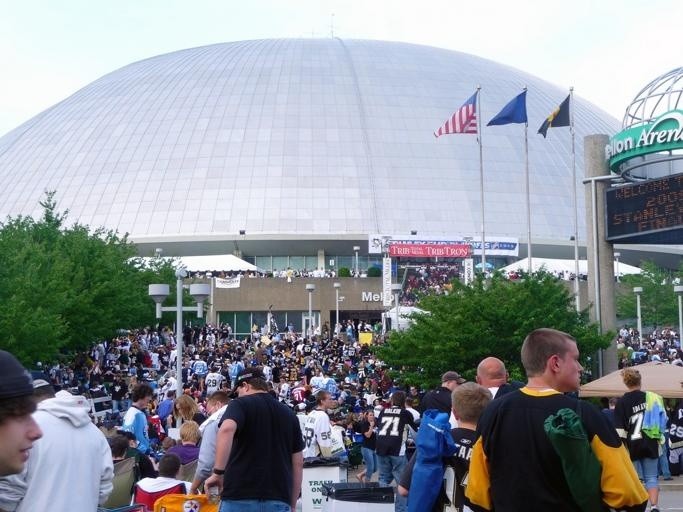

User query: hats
[442,371,466,384]
[0,351,33,398]
[227,368,267,399]
[31,371,51,389]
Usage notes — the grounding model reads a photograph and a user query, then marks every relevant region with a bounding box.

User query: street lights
[610,251,681,359]
[305,283,342,337]
[148,266,211,398]
[352,245,361,273]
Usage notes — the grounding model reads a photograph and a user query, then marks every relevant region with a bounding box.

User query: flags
[537,94,570,139]
[487,91,528,125]
[433,91,477,137]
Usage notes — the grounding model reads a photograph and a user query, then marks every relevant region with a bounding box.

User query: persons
[294,357,511,512]
[49,321,421,409]
[0,348,43,477]
[403,262,462,306]
[617,322,681,363]
[109,368,305,512]
[638,407,644,423]
[608,369,682,511]
[1,371,114,512]
[462,328,648,512]
[192,268,365,279]
